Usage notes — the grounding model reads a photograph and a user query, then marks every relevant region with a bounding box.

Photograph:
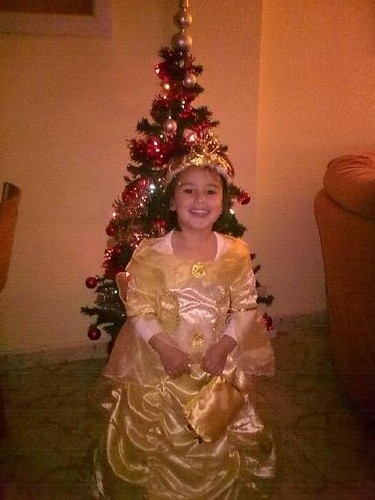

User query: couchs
[311,154,374,416]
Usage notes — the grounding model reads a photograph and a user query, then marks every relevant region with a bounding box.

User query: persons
[83,122,277,500]
